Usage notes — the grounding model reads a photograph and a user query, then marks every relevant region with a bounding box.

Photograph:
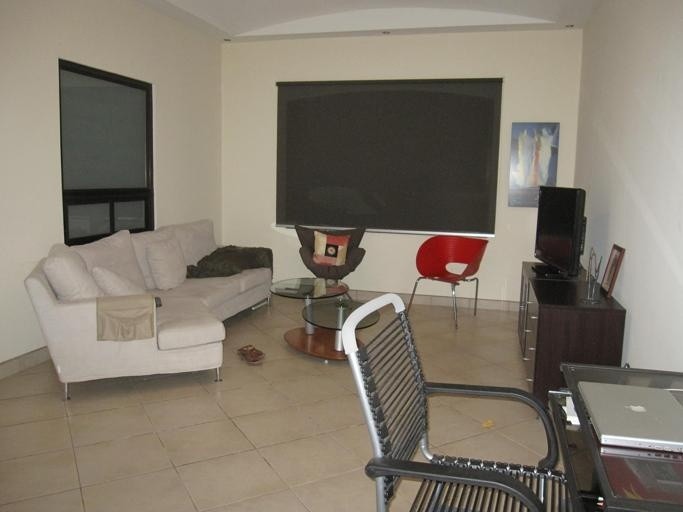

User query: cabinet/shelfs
[519,261,625,410]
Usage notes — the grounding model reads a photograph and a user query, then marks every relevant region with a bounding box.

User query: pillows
[43,220,219,302]
[313,231,350,267]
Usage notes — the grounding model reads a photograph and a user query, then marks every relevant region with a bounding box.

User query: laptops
[578,381,683,453]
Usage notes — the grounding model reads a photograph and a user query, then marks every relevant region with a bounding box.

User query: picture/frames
[600,245,625,299]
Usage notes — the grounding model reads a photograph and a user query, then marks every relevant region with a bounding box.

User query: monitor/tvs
[532,185,586,279]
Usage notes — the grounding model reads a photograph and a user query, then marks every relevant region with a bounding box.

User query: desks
[547,363,683,512]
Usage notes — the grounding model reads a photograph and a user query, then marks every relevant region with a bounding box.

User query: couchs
[23,245,272,404]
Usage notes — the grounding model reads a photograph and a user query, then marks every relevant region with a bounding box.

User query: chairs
[342,294,581,512]
[294,224,366,280]
[405,235,488,331]
